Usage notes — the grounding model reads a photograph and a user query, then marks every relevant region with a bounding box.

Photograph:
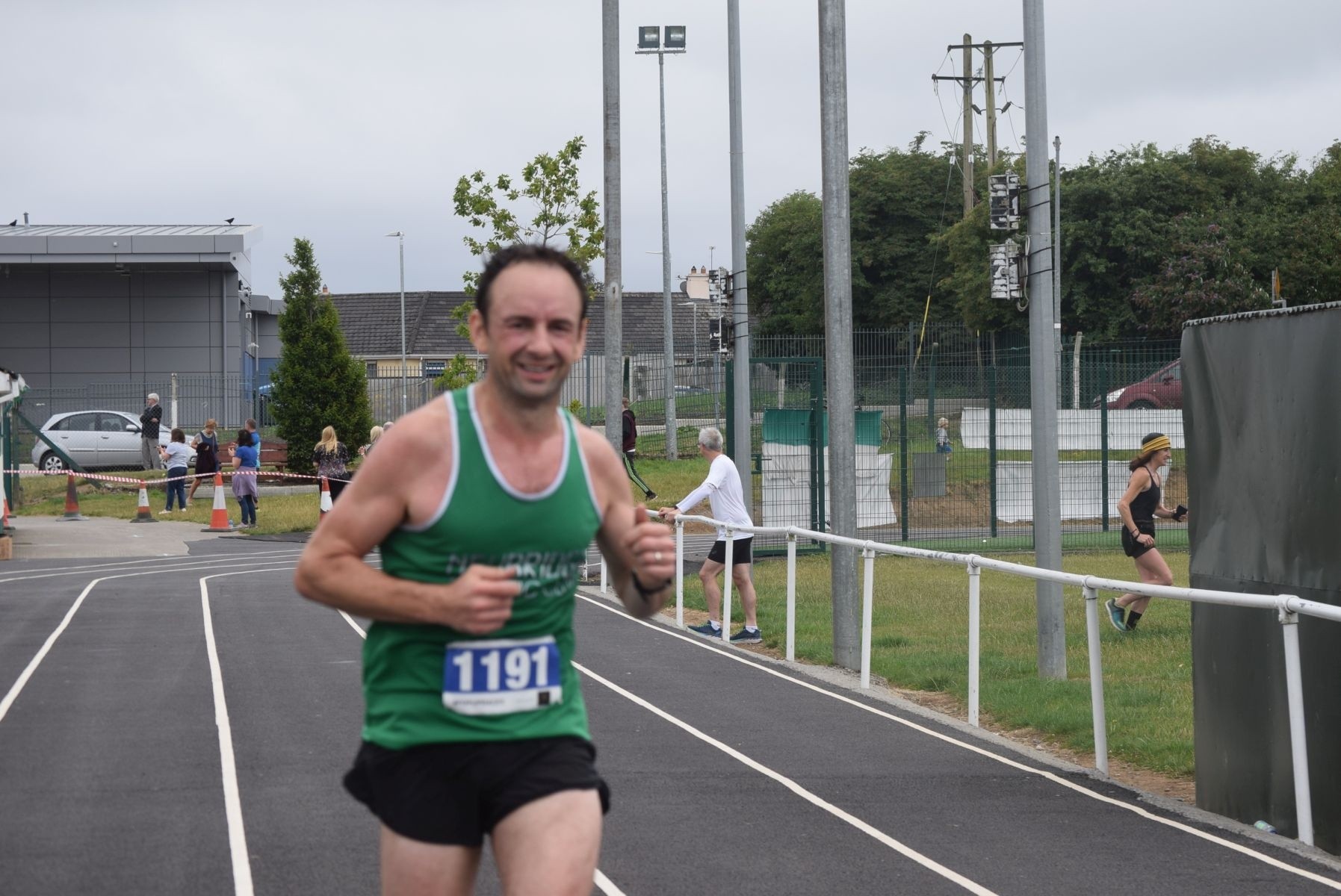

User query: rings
[653,551,664,562]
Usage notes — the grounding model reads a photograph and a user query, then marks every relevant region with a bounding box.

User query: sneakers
[254,506,260,511]
[686,620,722,637]
[730,627,762,644]
[236,522,248,528]
[645,492,658,502]
[249,523,258,528]
[158,508,172,515]
[1105,597,1128,633]
[179,508,186,512]
[185,499,193,507]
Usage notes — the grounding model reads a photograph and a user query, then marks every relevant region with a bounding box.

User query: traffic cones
[129,477,159,523]
[55,470,90,521]
[0,503,13,538]
[200,472,239,533]
[0,484,18,518]
[1,491,16,530]
[318,477,335,527]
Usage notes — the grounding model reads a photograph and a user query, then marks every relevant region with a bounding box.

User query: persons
[657,427,763,644]
[312,424,350,505]
[1103,432,1188,632]
[358,424,384,459]
[621,396,657,501]
[935,417,956,462]
[137,391,264,530]
[292,246,679,896]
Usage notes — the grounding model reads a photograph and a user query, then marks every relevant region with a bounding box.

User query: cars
[1093,357,1183,411]
[32,411,199,477]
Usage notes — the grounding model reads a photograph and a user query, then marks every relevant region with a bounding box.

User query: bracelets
[361,453,364,456]
[1133,531,1141,540]
[1131,527,1138,535]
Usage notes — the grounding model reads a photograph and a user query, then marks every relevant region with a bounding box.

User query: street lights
[384,230,408,420]
[635,23,687,460]
[676,301,699,395]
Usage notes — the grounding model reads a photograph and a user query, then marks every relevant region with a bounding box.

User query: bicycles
[851,391,891,449]
